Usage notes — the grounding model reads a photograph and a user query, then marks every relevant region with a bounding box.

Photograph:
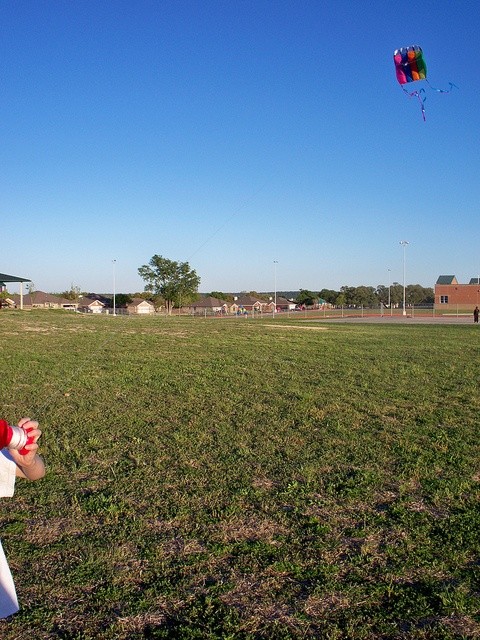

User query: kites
[394,44,456,121]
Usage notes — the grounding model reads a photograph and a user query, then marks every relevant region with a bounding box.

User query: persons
[0,415,46,621]
